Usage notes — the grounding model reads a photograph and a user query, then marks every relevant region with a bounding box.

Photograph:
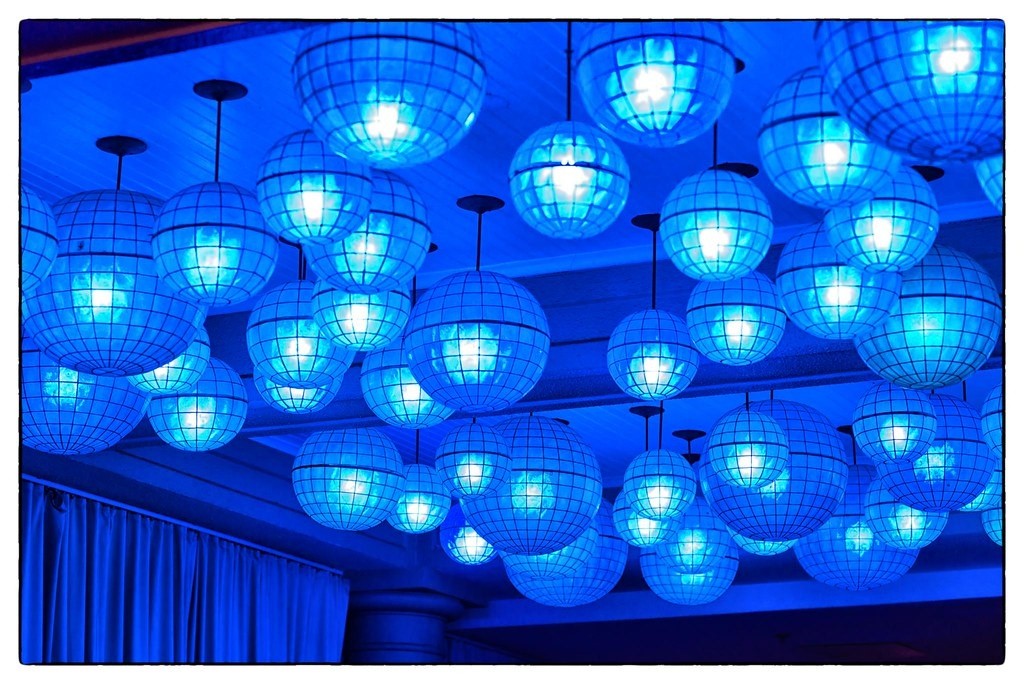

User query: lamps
[20,19,1006,608]
